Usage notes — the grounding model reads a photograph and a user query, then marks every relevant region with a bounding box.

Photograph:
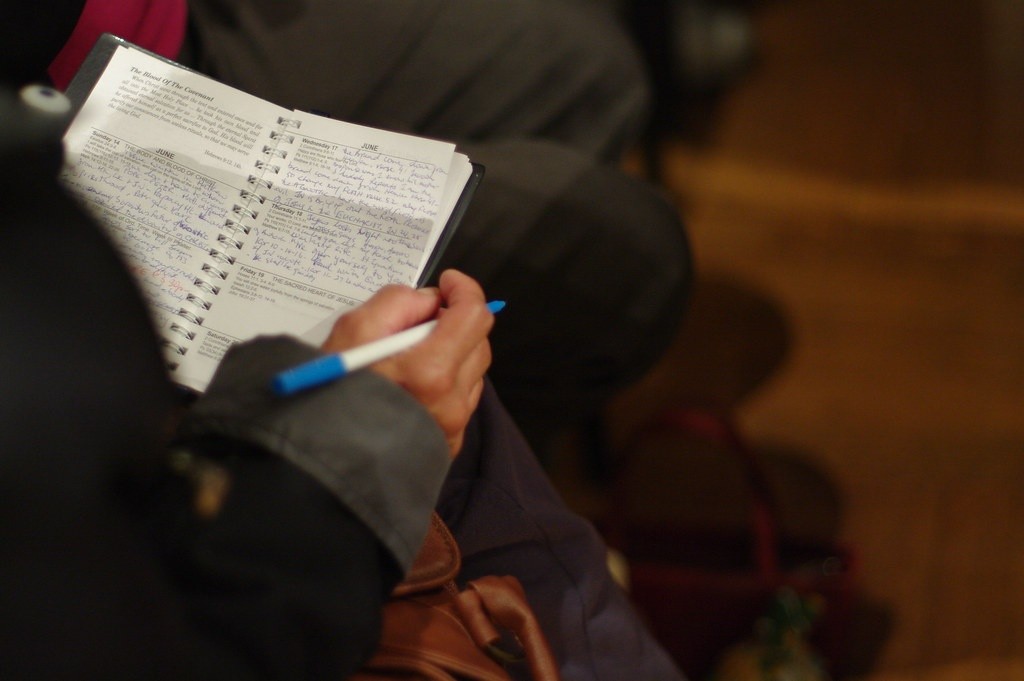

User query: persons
[0,0,697,680]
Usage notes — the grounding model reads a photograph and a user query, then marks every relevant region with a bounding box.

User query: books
[55,31,486,406]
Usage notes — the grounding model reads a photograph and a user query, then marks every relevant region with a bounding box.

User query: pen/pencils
[274,300,509,395]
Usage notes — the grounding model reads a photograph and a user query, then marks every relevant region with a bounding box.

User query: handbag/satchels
[350,510,557,681]
